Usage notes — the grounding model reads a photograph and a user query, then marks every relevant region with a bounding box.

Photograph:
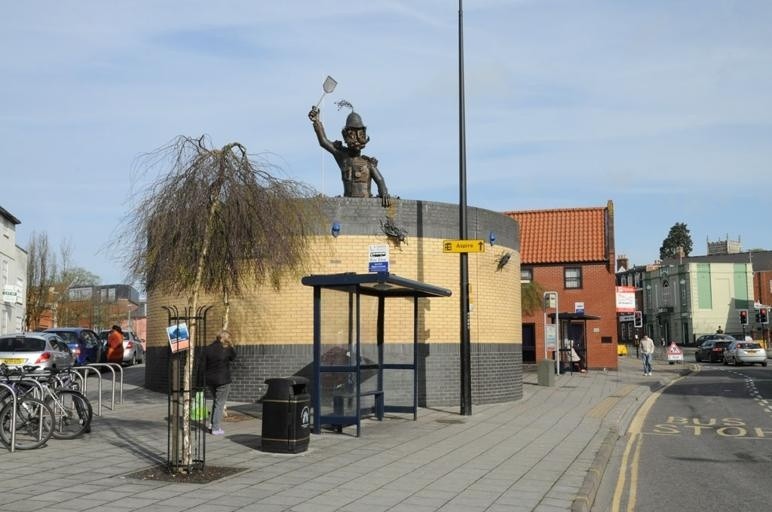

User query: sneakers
[208,423,224,434]
[643,372,653,376]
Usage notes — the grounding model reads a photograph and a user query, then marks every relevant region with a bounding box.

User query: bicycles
[0,363,92,450]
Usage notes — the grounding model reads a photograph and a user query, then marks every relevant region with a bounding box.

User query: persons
[637,333,655,377]
[308,105,392,208]
[715,325,723,334]
[196,331,237,435]
[106,325,125,372]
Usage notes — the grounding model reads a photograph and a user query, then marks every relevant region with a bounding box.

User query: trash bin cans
[538,360,555,386]
[261,376,310,454]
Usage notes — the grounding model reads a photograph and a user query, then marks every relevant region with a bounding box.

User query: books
[165,322,190,354]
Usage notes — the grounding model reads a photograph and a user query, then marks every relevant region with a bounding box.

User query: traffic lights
[759,308,769,324]
[634,310,643,328]
[740,310,749,326]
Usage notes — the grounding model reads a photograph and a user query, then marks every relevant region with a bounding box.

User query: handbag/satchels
[192,392,208,420]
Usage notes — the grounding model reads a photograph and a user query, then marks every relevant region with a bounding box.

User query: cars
[722,341,768,367]
[97,329,145,366]
[1,331,74,371]
[695,339,733,362]
[696,334,736,348]
[42,328,106,373]
